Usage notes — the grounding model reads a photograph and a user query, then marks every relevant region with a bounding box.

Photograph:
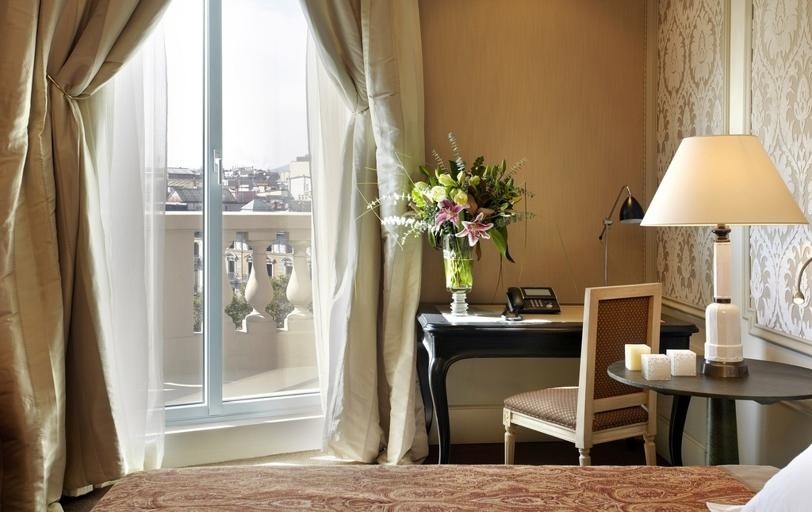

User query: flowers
[349,127,536,293]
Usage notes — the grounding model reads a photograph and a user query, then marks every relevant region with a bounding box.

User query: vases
[439,232,476,316]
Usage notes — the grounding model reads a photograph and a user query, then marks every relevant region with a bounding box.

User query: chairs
[500,282,663,464]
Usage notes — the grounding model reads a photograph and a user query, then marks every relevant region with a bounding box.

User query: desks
[606,356,812,466]
[416,300,699,466]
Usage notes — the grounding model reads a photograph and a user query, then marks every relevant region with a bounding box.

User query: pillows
[704,443,811,512]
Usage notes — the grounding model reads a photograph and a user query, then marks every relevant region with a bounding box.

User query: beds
[86,463,811,512]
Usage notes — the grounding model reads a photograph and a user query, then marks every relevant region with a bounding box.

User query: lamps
[640,134,810,380]
[598,184,644,285]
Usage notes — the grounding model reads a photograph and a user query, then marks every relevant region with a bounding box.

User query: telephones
[506,287,560,314]
